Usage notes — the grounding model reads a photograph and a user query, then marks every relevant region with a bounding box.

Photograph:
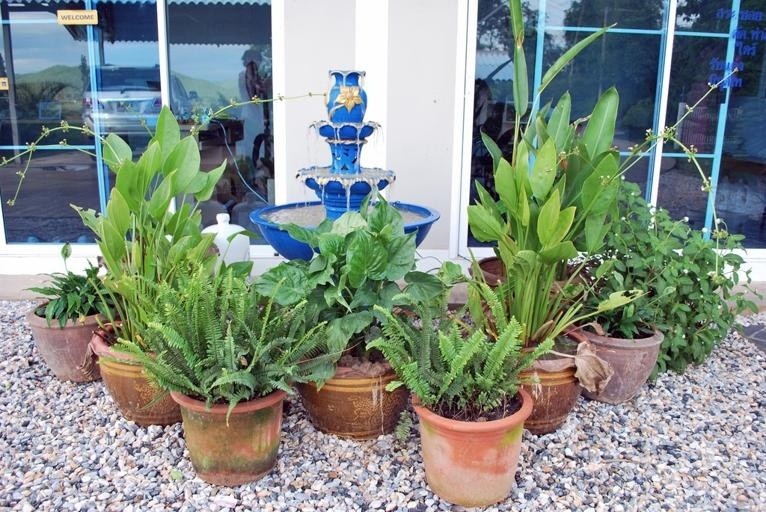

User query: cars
[82,64,199,154]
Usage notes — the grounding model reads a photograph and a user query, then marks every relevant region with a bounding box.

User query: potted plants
[367,1,762,506]
[3,92,447,487]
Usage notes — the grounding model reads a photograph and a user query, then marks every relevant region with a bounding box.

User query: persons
[238,50,272,167]
